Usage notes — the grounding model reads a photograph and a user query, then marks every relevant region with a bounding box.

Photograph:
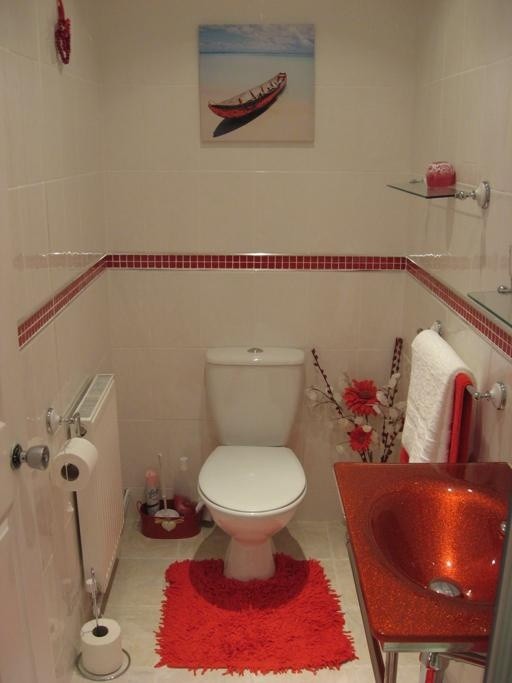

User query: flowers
[308,337,406,462]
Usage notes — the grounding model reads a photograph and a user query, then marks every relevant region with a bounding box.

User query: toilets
[198,348,308,583]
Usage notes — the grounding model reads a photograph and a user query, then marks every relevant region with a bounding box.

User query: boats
[208,72,288,124]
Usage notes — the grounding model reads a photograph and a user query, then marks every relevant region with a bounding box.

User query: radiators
[70,374,124,592]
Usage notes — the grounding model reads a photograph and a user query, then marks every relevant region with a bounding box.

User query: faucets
[499,519,509,537]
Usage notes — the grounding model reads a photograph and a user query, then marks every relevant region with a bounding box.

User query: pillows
[400,329,478,463]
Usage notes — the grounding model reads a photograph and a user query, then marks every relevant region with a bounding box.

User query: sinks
[369,485,506,607]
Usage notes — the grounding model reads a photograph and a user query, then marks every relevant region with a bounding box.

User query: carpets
[153,552,359,676]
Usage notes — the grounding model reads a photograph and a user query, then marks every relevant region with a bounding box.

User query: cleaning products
[174,455,199,515]
